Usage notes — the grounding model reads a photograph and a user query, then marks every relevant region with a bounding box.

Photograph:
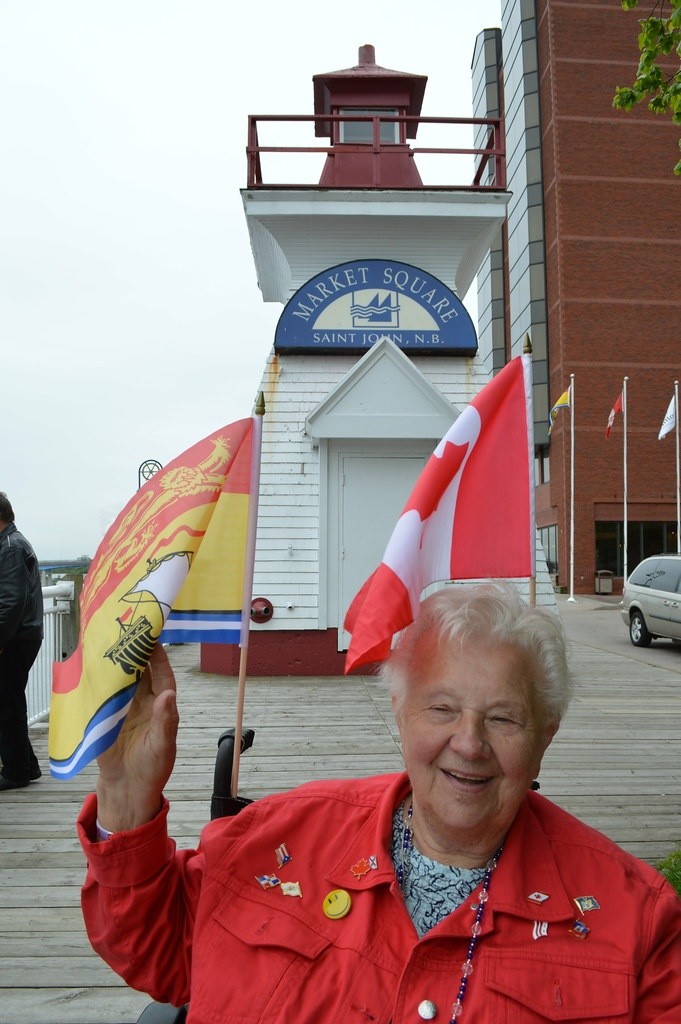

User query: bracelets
[95,822,112,840]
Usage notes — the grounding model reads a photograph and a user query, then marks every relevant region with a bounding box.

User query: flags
[48,419,253,780]
[548,385,571,435]
[344,357,533,677]
[606,390,623,440]
[658,395,676,440]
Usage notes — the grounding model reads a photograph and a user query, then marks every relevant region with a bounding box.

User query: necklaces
[400,797,502,1024]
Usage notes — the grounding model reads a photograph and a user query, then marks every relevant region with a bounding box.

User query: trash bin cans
[595,570,615,595]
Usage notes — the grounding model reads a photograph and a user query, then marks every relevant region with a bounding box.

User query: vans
[618,551,681,646]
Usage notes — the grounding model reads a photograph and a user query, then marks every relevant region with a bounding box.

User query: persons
[0,492,44,790]
[83,583,681,1024]
